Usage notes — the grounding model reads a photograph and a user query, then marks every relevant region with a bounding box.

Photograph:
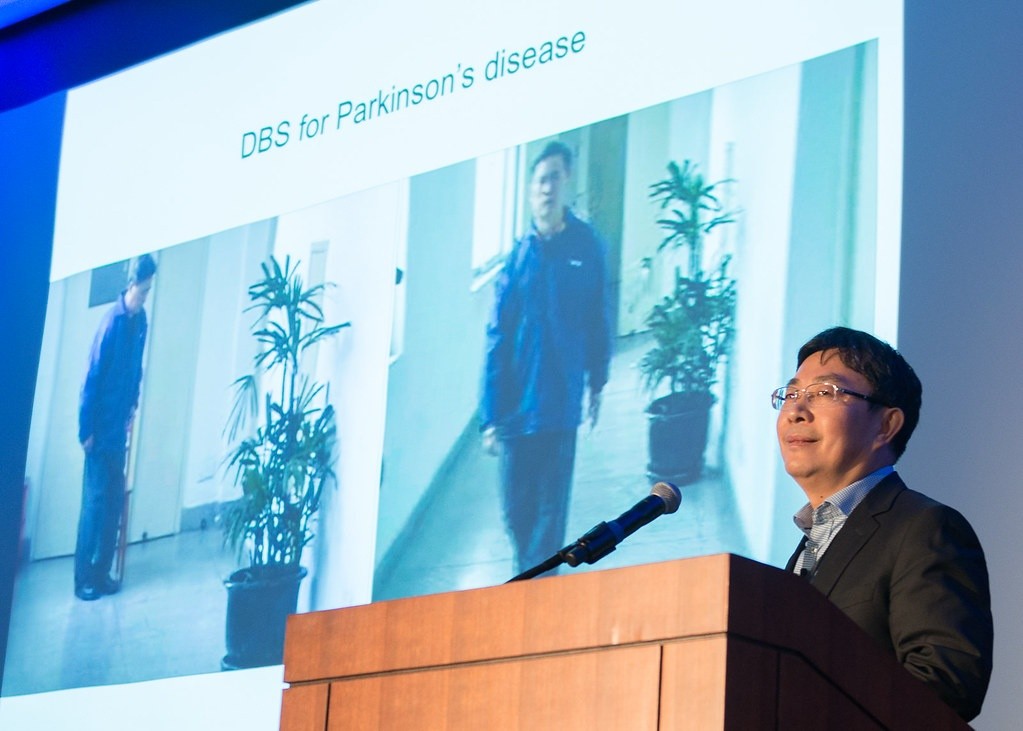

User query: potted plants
[212,250,357,671]
[627,157,743,489]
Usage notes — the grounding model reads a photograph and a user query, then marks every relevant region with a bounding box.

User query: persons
[758,321,993,725]
[475,141,614,579]
[69,253,159,604]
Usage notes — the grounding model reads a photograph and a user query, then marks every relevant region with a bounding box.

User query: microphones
[567,480,682,565]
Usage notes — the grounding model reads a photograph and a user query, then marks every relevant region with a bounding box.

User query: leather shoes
[74,586,98,600]
[98,575,119,594]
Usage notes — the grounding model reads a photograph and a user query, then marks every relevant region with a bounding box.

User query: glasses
[771,383,871,411]
